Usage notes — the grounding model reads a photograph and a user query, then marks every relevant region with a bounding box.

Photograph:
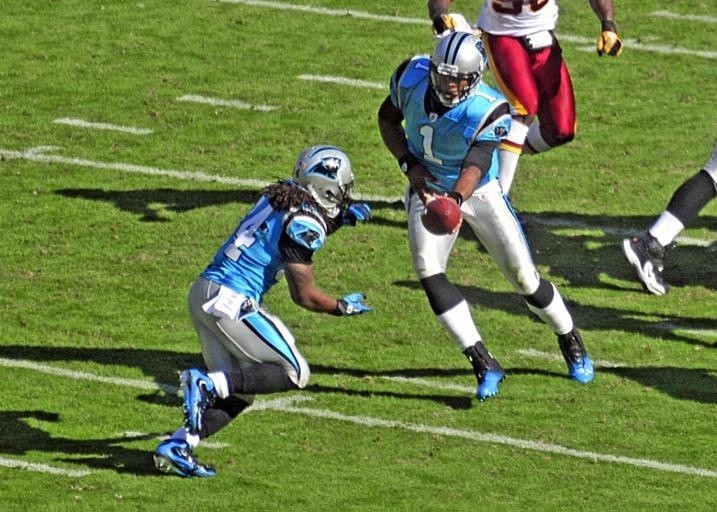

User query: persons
[151,143,374,479]
[427,0,625,231]
[620,148,717,297]
[376,31,594,401]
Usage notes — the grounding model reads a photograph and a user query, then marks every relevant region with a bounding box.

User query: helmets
[429,31,487,108]
[290,145,355,209]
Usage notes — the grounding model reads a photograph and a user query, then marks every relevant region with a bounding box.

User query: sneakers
[554,326,595,383]
[621,229,678,295]
[178,368,218,435]
[153,438,216,477]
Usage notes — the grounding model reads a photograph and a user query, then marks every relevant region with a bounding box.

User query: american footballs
[421,199,460,234]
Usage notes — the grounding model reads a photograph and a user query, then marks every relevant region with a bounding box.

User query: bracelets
[397,153,419,175]
[448,192,464,207]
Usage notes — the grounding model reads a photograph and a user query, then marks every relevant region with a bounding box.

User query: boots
[462,341,505,402]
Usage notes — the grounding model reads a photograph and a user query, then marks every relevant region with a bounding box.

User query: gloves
[340,199,372,226]
[432,13,482,40]
[597,28,623,57]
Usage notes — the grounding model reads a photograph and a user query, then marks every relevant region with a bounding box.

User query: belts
[327,293,373,317]
